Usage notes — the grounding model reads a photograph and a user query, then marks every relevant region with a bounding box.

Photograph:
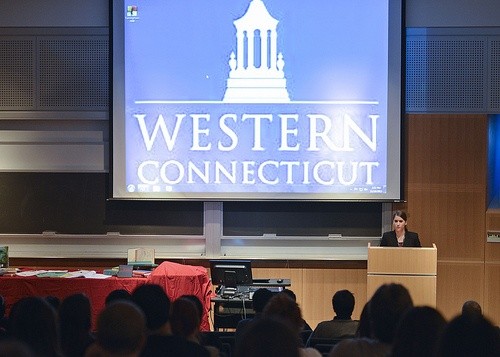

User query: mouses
[277,279,283,283]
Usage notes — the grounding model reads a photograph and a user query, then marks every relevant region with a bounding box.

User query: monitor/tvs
[209,260,253,296]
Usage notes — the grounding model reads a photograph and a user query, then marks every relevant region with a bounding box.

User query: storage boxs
[127,248,154,263]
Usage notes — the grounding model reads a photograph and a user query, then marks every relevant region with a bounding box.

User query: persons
[378,210,421,247]
[463,301,482,315]
[306,289,361,348]
[330,282,413,357]
[439,313,500,357]
[231,287,322,357]
[106,283,220,357]
[87,302,146,357]
[391,305,445,357]
[0,291,94,357]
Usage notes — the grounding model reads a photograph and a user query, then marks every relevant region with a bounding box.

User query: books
[66,270,111,279]
[0,246,9,267]
[0,247,159,279]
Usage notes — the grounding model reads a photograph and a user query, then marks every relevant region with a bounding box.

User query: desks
[0,261,216,332]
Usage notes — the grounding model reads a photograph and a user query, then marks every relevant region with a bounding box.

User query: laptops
[127,248,159,267]
